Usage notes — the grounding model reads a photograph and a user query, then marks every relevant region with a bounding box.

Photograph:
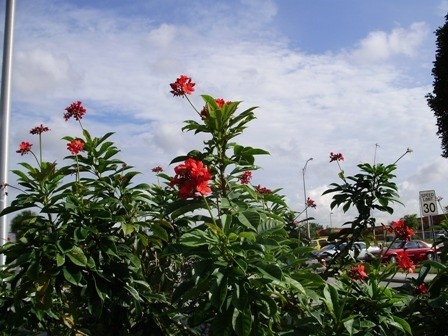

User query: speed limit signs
[419,191,438,217]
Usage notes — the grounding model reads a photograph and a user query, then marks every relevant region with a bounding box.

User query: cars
[308,237,338,257]
[315,241,380,265]
[378,239,440,267]
